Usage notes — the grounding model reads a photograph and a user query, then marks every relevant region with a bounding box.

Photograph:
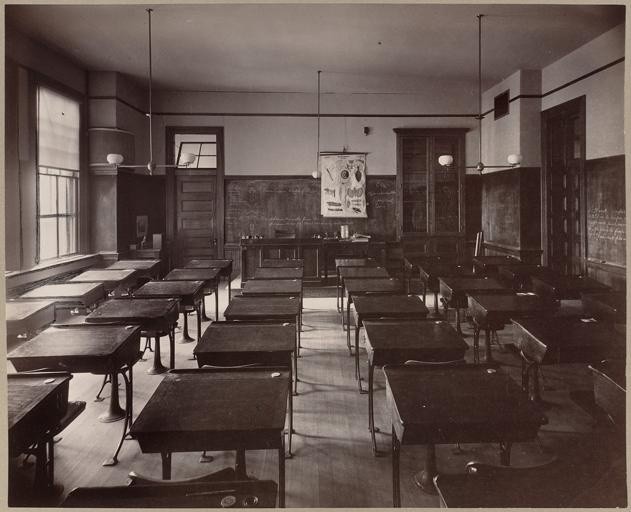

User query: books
[352,238,369,242]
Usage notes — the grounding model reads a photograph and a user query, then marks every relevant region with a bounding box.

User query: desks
[5,238,626,508]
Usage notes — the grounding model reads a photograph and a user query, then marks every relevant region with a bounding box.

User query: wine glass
[249,223,255,239]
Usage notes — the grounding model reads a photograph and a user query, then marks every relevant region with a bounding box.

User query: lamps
[437,16,522,173]
[105,8,197,175]
[312,71,322,180]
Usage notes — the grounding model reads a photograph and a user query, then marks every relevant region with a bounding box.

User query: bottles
[141,236,148,247]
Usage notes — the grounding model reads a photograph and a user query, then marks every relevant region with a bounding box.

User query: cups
[242,233,265,241]
[314,224,350,240]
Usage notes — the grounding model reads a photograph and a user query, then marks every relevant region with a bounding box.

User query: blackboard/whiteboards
[584,154,627,269]
[224,179,396,244]
